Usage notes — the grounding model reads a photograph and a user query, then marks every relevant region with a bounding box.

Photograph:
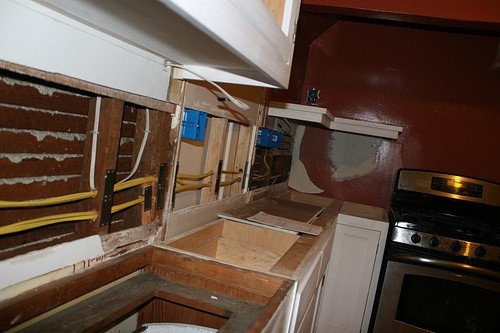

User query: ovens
[367,242,500,333]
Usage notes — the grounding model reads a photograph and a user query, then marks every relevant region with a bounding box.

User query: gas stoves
[387,168,500,267]
